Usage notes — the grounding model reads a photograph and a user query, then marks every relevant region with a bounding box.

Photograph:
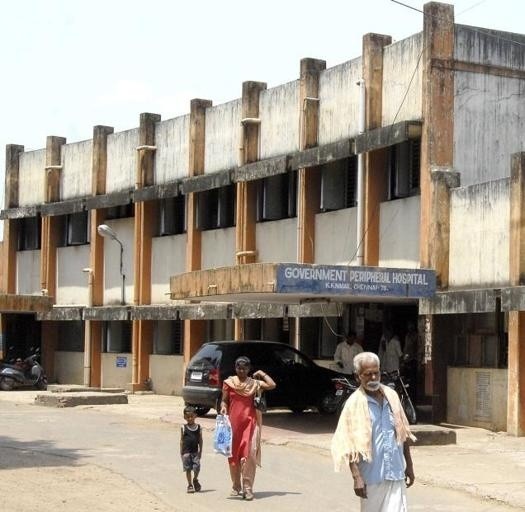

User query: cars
[183,340,354,418]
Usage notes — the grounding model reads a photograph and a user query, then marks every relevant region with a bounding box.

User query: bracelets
[263,373,267,378]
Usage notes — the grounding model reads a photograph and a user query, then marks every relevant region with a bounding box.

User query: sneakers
[193,478,201,492]
[242,490,254,501]
[231,484,242,496]
[187,484,194,494]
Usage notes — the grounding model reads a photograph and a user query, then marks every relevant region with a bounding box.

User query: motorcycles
[0,344,48,391]
[330,353,420,425]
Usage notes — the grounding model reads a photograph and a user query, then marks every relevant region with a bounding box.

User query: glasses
[357,369,380,378]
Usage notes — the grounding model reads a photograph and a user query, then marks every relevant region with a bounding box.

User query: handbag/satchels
[212,414,233,458]
[255,390,267,414]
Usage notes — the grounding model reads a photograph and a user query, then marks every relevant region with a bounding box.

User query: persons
[220,355,277,501]
[378,323,420,372]
[330,351,418,512]
[333,330,365,369]
[179,406,203,492]
